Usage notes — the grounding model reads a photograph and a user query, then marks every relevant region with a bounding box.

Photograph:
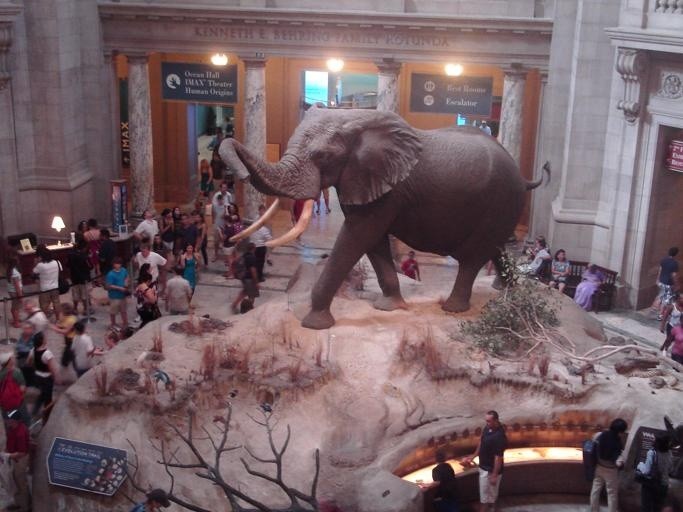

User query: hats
[145,488,171,508]
[0,351,13,366]
[8,410,24,421]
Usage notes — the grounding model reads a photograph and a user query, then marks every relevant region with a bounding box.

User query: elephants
[217,102,550,330]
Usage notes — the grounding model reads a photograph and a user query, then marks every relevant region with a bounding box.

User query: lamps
[51,215,66,246]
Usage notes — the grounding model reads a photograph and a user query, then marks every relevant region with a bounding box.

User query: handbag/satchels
[56,261,70,295]
[583,440,598,474]
[635,449,661,488]
[137,303,162,322]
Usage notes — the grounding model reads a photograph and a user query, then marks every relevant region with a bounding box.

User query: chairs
[518,244,618,313]
[7,232,37,246]
